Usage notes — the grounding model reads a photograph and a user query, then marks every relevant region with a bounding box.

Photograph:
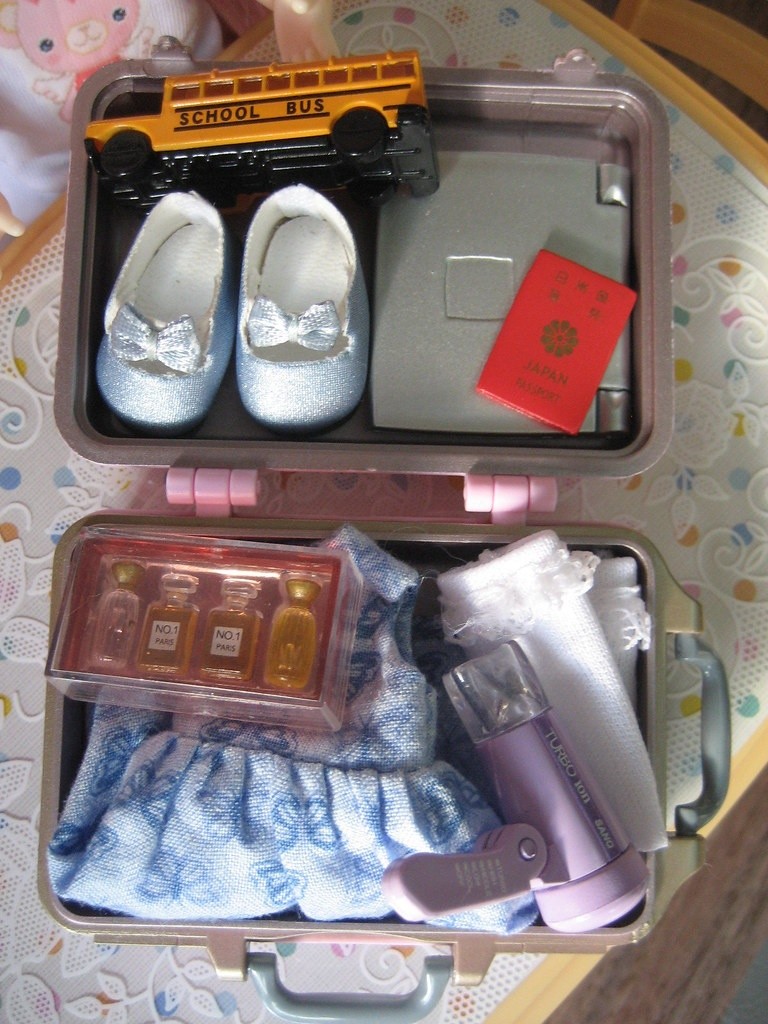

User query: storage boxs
[44,515,366,735]
[41,65,670,1023]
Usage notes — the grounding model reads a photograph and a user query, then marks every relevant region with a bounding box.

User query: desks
[0,0,767,1023]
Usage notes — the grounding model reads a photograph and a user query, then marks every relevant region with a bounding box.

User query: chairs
[612,4,768,111]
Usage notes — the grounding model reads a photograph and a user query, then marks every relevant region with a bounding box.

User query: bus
[80,46,441,207]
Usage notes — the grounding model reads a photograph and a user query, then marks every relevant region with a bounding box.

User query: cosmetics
[89,562,322,691]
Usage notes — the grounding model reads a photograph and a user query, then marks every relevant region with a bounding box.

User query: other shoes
[96,191,235,429]
[234,182,370,427]
